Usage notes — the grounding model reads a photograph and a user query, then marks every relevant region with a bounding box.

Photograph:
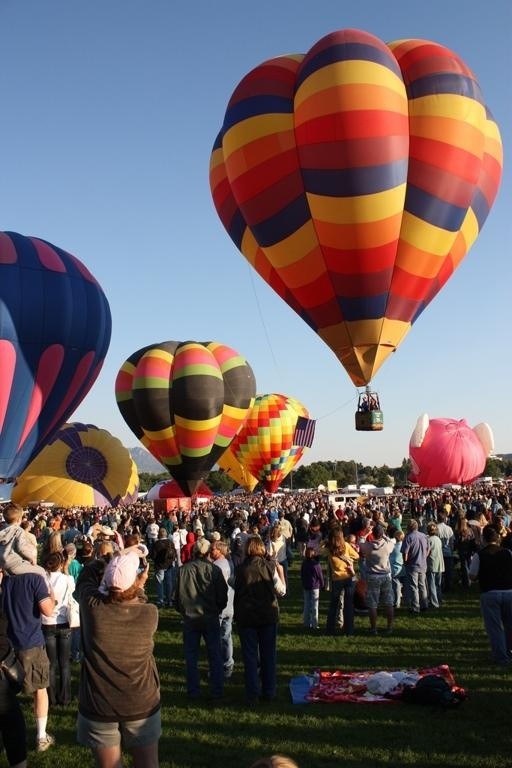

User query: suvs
[419,490,440,502]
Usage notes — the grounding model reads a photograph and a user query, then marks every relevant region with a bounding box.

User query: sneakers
[36,736,53,752]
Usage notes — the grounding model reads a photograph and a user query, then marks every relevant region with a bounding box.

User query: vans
[371,494,410,507]
[318,491,364,514]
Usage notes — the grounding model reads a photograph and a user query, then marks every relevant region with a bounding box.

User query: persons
[469,519,512,670]
[73,544,166,765]
[231,533,288,699]
[0,567,30,766]
[326,477,511,633]
[206,534,236,675]
[191,489,334,627]
[1,494,207,664]
[361,396,378,412]
[2,573,58,752]
[172,540,227,703]
[37,552,81,705]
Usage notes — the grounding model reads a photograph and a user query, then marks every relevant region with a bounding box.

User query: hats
[193,540,208,554]
[96,526,115,536]
[104,551,139,592]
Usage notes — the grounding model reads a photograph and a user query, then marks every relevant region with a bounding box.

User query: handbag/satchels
[402,685,442,714]
[0,651,26,683]
[416,675,454,701]
